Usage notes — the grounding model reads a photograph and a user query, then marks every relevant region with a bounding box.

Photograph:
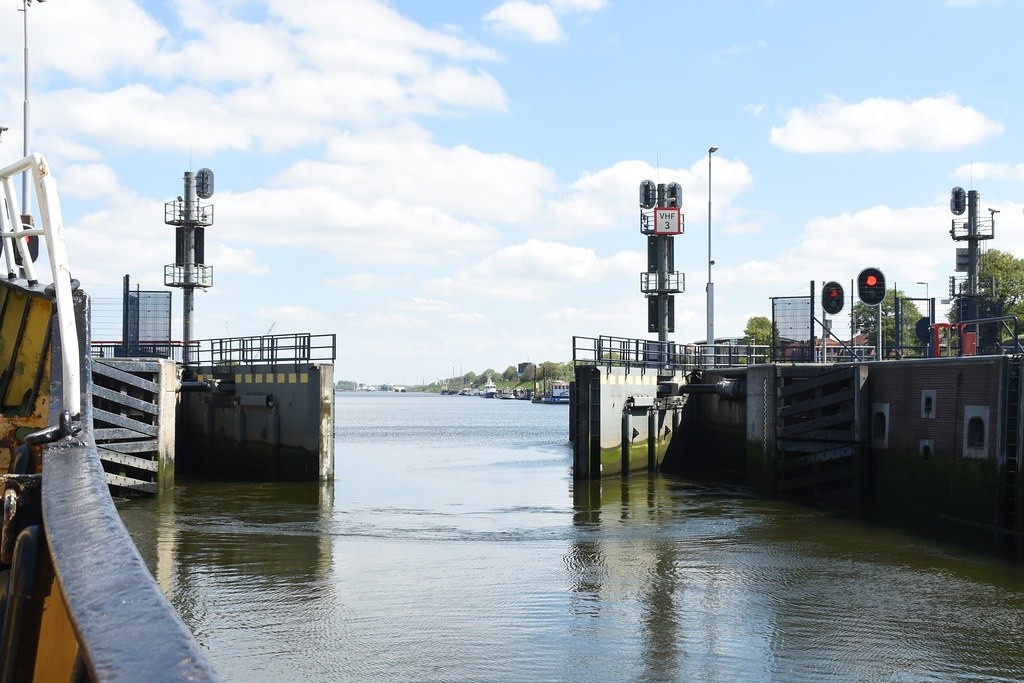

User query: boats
[355,362,573,407]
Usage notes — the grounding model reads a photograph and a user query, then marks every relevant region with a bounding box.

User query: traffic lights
[822,282,844,313]
[858,267,886,306]
